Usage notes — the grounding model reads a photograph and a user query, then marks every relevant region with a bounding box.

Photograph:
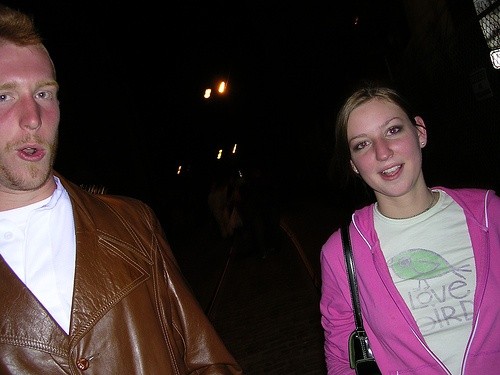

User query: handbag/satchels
[349,329,383,375]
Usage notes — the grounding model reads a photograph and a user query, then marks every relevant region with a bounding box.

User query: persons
[1,11,247,375]
[318,81,500,375]
[207,177,258,267]
